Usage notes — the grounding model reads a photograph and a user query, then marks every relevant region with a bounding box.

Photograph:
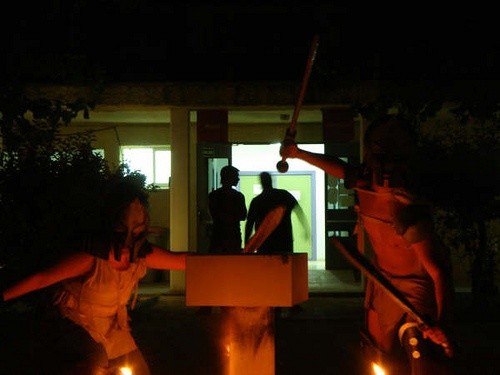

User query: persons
[0,173,200,375]
[207,166,249,254]
[244,172,311,255]
[281,115,446,375]
